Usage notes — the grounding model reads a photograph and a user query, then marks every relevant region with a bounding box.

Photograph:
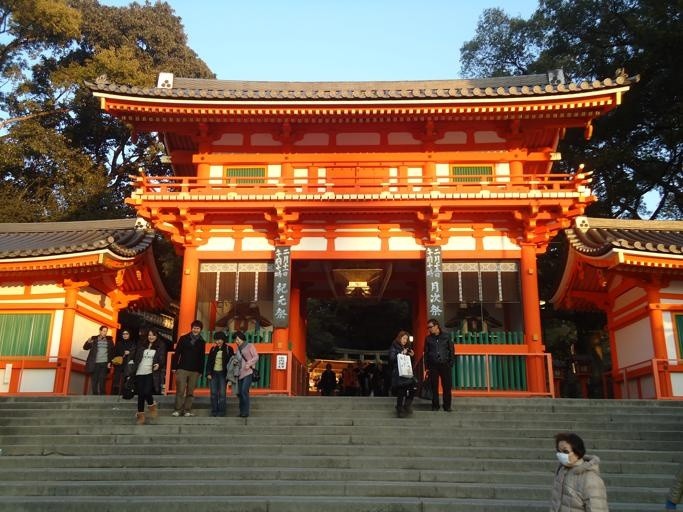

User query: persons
[549,432,609,512]
[423,318,455,411]
[663,466,683,511]
[82,325,165,424]
[232,330,259,417]
[205,330,235,417]
[171,319,207,417]
[389,330,416,414]
[320,358,392,397]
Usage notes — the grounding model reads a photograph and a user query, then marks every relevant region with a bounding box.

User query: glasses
[557,449,570,454]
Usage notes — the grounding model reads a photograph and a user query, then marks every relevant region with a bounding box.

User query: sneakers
[172,412,191,417]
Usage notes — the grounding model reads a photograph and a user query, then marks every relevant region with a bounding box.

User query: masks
[555,452,569,466]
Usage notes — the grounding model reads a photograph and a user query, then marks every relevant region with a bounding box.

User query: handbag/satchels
[123,378,136,399]
[252,369,259,381]
[397,353,414,378]
[111,356,123,365]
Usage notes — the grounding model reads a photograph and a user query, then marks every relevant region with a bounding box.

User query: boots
[147,401,157,419]
[396,398,412,413]
[136,412,145,425]
